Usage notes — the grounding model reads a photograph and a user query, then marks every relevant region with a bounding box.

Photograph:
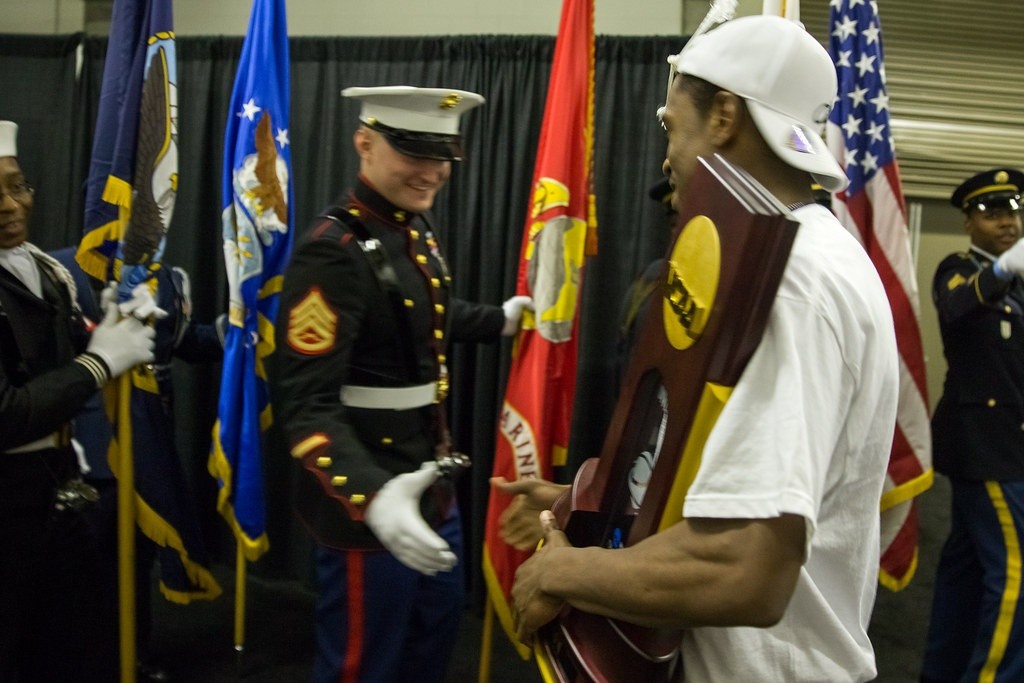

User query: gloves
[100,281,168,320]
[502,296,536,336]
[994,238,1024,281]
[86,301,156,379]
[363,469,458,577]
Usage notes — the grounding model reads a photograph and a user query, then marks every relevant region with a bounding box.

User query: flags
[480,0,599,662]
[829,0,935,593]
[74,0,224,605]
[205,0,296,563]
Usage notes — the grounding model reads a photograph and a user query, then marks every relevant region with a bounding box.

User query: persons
[489,13,901,683]
[0,118,259,683]
[268,83,536,683]
[918,168,1024,683]
[613,176,837,452]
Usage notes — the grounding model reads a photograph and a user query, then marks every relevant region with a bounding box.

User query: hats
[0,120,18,157]
[341,87,485,161]
[673,15,849,193]
[951,168,1024,215]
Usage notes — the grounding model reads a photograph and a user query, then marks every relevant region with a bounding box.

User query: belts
[2,426,72,454]
[338,381,438,410]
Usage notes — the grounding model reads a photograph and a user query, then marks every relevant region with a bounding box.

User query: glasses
[0,179,33,199]
